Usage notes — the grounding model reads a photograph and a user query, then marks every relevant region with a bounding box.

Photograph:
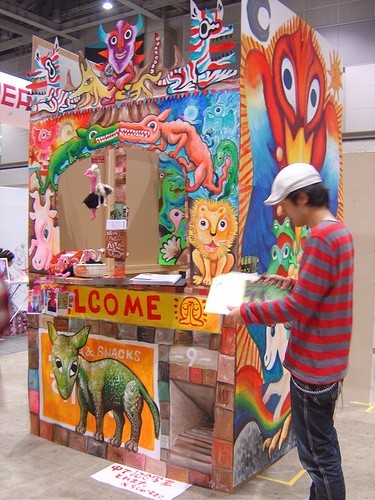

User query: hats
[263,163,322,206]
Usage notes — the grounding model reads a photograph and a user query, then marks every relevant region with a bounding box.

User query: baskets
[73,249,106,278]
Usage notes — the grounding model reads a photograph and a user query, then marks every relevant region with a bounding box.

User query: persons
[225,162,354,498]
[0,247,16,282]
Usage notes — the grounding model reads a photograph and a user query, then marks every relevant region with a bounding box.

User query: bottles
[105,220,128,280]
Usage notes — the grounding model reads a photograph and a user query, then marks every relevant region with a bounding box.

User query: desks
[10,281,29,322]
